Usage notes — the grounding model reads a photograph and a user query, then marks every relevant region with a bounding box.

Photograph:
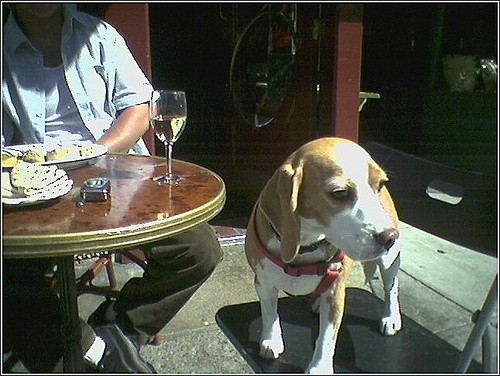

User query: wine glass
[147,90,187,186]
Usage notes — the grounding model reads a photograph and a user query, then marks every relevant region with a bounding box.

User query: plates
[1,167,74,206]
[1,141,108,170]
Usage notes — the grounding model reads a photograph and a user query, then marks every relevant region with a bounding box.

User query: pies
[1,160,73,199]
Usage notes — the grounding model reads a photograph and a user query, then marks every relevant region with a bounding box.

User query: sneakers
[87,302,140,352]
[92,323,156,374]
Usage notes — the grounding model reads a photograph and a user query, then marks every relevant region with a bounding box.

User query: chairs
[27,251,161,345]
[216,142,498,374]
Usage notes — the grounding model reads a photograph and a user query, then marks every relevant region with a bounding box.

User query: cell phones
[80,178,110,203]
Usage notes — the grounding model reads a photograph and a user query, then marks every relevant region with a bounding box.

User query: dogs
[245,137,402,374]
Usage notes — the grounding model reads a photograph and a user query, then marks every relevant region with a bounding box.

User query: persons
[2,2,223,375]
